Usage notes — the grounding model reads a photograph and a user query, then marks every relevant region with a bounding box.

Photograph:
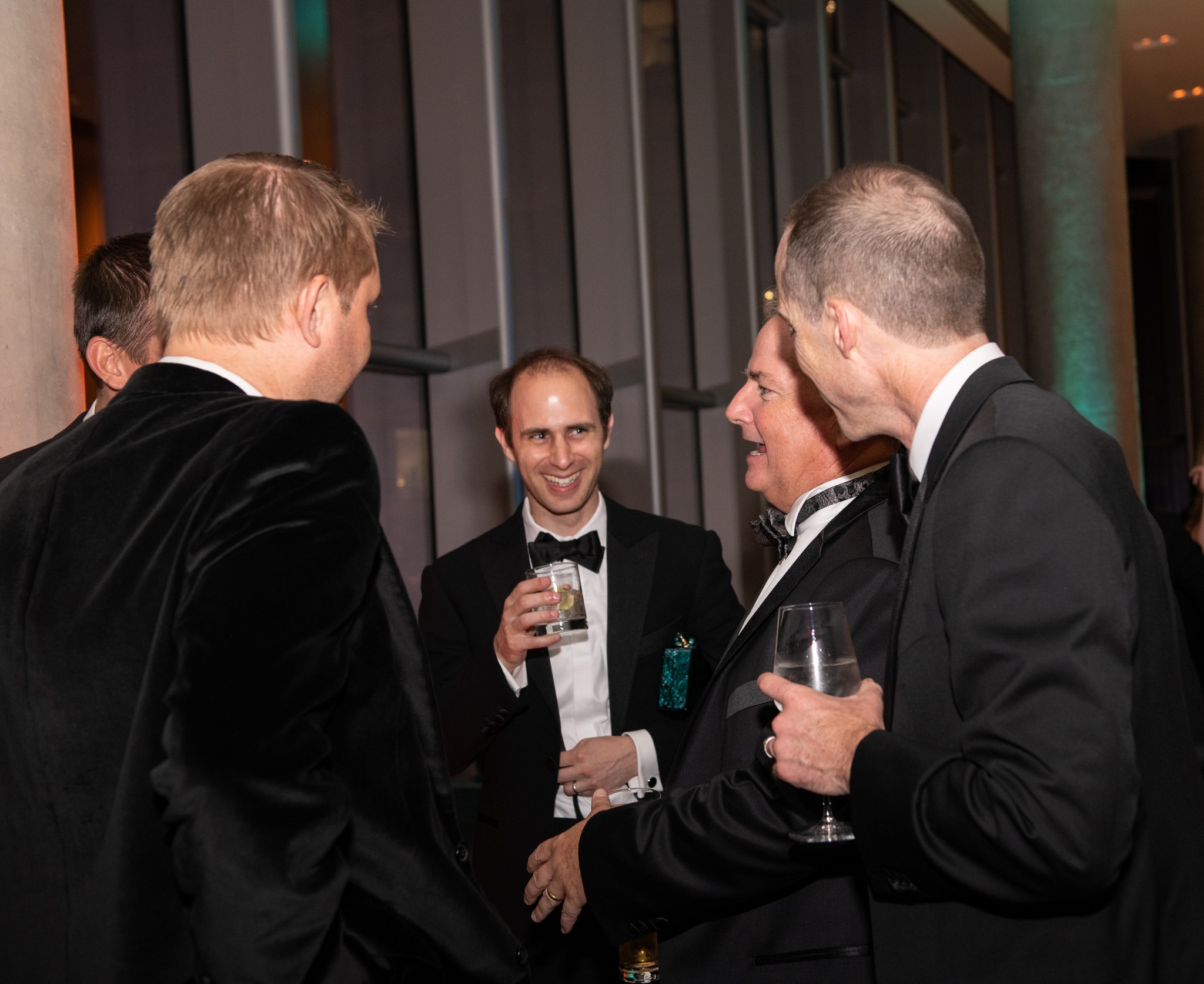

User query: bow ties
[748,465,890,560]
[528,531,605,574]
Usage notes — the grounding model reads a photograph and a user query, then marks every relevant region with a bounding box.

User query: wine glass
[773,602,857,841]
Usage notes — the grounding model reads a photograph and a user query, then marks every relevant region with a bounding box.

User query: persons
[753,164,1203,983]
[528,309,877,980]
[5,149,525,983]
[422,349,752,982]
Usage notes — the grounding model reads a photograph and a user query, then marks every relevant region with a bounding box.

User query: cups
[618,931,659,983]
[525,563,589,637]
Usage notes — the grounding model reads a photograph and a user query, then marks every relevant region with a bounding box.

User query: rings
[572,780,581,795]
[547,886,565,901]
[763,736,775,758]
